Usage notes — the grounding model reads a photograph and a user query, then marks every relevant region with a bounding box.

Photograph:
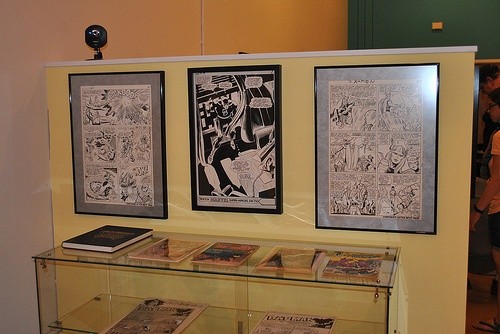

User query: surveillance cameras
[85,25,108,51]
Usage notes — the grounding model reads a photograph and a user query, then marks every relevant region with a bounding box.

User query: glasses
[489,102,498,112]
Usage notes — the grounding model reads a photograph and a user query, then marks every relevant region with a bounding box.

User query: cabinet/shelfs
[31,230,401,334]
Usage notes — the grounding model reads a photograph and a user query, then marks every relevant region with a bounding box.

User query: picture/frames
[314,62,440,235]
[67,71,168,220]
[187,64,283,214]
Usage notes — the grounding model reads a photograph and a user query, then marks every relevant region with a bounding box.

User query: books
[63,237,152,259]
[61,225,154,252]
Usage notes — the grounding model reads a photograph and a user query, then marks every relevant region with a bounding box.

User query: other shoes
[472,318,500,334]
